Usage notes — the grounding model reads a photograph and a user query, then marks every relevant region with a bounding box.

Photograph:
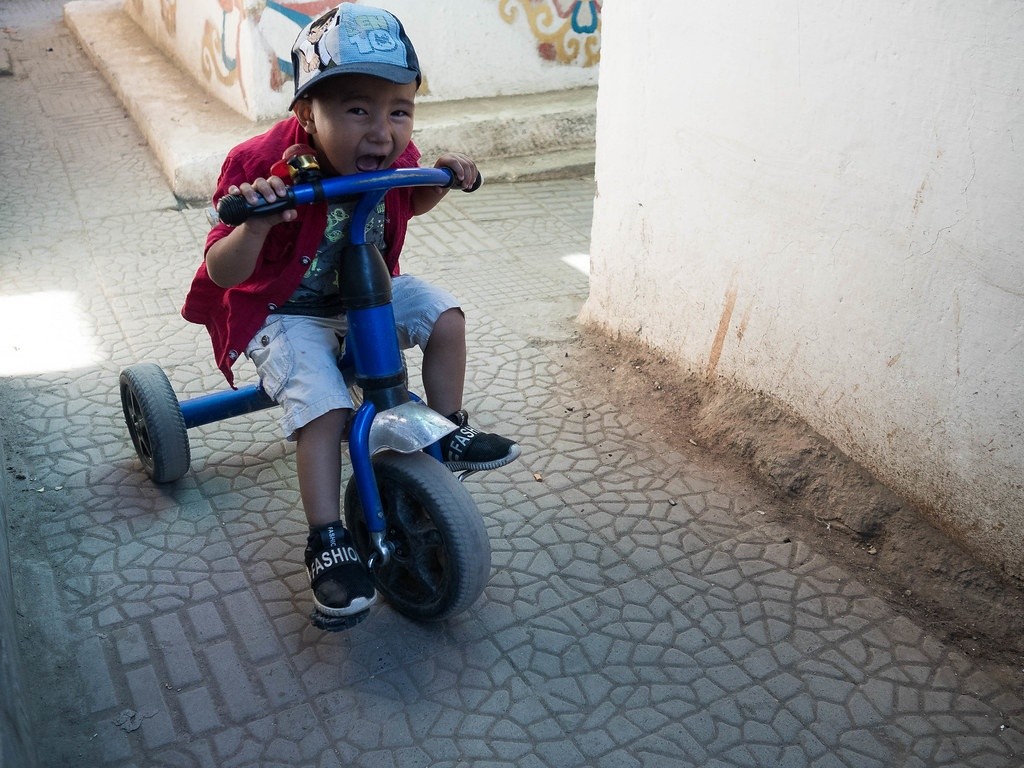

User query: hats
[288,2,422,114]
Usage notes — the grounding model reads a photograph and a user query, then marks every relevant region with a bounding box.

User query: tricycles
[118,143,491,632]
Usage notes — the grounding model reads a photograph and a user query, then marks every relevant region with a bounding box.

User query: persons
[182,2,521,616]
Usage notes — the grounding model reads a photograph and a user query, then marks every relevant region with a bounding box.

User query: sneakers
[422,408,522,472]
[303,523,379,616]
